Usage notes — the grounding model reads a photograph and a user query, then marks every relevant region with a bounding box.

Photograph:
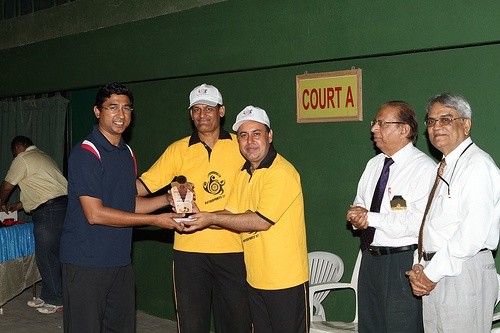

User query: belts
[367,244,418,256]
[31,195,68,212]
[423,248,487,261]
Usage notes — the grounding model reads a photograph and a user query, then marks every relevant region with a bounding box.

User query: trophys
[171,176,198,222]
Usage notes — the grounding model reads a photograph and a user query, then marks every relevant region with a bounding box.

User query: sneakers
[36,303,64,314]
[27,296,45,308]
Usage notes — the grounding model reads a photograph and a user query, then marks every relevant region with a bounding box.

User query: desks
[0,221,43,315]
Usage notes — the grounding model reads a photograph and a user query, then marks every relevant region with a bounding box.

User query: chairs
[307,248,361,333]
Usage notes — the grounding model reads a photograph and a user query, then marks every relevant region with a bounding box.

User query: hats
[231,105,270,131]
[188,82,223,110]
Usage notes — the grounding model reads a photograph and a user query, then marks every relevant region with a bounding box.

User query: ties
[417,158,446,263]
[360,157,395,253]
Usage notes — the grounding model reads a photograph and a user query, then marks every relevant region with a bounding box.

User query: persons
[136,83,251,333]
[346,100,438,333]
[0,138,69,314]
[406,92,500,332]
[182,105,310,333]
[59,81,185,333]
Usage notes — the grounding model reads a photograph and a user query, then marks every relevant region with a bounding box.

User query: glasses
[423,117,466,127]
[102,106,133,115]
[370,119,407,126]
